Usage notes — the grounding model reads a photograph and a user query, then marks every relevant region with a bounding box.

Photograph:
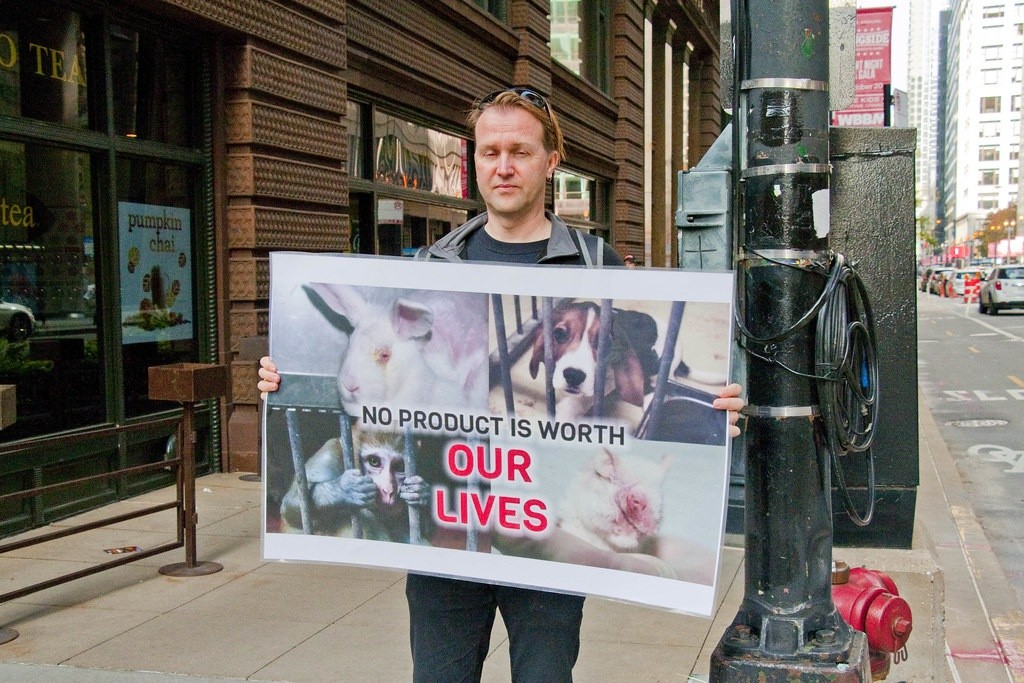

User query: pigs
[560,446,675,554]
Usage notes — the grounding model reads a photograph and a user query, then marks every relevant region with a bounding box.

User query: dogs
[529,301,644,427]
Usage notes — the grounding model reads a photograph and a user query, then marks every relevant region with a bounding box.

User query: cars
[917,266,994,297]
[0,299,35,342]
[979,265,1024,315]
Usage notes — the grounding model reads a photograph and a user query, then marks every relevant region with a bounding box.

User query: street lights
[974,232,983,265]
[1003,220,1016,266]
[991,226,1001,266]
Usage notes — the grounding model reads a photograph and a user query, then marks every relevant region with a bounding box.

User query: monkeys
[280,424,431,545]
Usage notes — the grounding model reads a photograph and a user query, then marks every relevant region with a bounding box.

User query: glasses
[478,89,551,122]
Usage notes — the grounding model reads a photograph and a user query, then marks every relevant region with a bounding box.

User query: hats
[624,254,636,263]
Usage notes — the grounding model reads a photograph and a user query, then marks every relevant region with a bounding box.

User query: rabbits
[309,282,489,436]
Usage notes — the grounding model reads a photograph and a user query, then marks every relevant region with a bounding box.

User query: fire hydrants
[832,560,913,681]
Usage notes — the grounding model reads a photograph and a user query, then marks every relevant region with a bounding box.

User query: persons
[80,260,98,357]
[257,87,748,683]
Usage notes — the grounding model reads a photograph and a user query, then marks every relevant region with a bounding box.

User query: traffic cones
[940,284,945,297]
[949,284,953,298]
